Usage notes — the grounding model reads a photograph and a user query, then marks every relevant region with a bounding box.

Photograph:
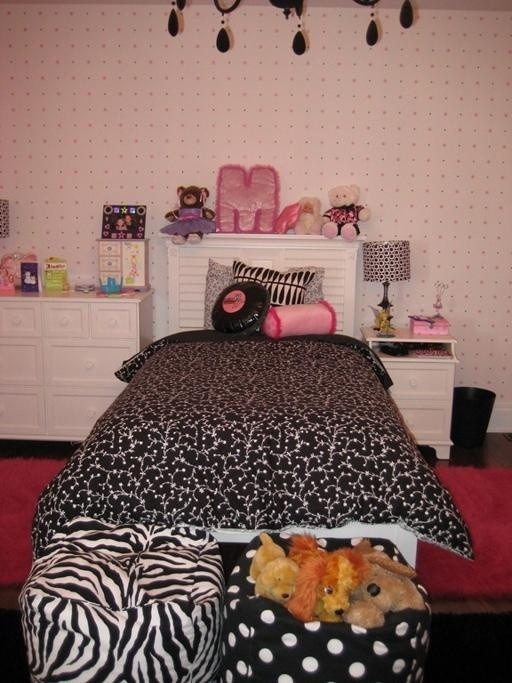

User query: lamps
[0,198,10,238]
[362,240,411,330]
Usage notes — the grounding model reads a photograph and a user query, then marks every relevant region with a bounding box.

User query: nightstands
[359,326,460,460]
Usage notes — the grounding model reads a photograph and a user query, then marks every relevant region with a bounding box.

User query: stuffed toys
[322,185,369,242]
[343,540,427,628]
[291,196,329,235]
[286,533,365,624]
[250,532,297,603]
[160,185,216,245]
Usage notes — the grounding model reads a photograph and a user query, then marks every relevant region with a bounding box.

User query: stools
[223,531,432,682]
[18,517,227,683]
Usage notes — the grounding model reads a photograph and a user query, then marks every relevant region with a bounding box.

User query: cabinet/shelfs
[96,237,151,291]
[0,291,154,444]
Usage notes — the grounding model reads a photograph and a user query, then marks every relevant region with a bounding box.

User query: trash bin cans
[451,386,496,447]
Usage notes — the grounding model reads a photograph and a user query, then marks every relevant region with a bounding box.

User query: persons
[115,218,127,230]
[124,215,137,232]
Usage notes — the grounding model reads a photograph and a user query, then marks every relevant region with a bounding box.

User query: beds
[31,231,419,571]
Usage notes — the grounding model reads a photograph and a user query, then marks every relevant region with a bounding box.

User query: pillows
[233,260,317,308]
[203,257,325,331]
[258,298,337,339]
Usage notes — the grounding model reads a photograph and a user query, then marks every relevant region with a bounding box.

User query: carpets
[0,457,511,602]
[0,605,512,682]
